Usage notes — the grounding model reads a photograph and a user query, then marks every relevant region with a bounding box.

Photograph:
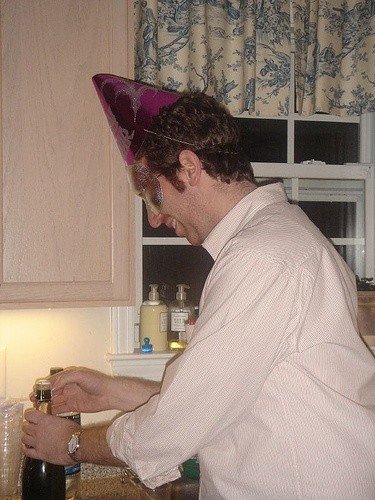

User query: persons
[22,71,375,499]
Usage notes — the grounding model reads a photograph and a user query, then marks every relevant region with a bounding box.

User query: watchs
[66,427,85,463]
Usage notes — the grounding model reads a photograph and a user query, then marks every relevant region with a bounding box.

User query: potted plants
[356,275,375,336]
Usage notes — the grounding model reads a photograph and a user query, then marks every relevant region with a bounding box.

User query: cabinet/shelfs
[0,0,139,309]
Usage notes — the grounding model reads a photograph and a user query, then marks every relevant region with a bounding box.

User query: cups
[0,398,25,497]
[185,324,194,345]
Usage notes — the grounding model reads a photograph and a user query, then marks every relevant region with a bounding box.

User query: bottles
[50,367,80,500]
[21,378,67,500]
